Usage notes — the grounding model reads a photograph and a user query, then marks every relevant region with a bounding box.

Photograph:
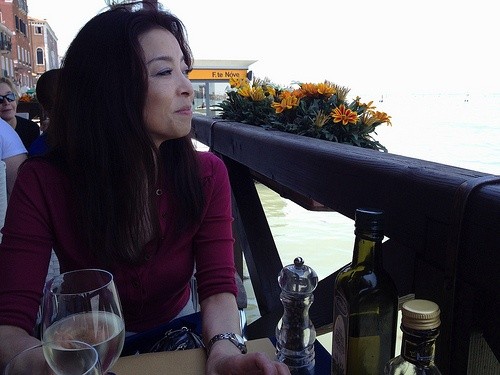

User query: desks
[107,338,332,375]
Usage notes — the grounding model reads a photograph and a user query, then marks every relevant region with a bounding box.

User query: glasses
[0,93,16,103]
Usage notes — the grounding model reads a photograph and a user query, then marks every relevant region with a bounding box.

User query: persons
[0,3,292,375]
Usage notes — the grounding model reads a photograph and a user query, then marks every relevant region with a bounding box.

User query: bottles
[382,299,446,375]
[330,205,398,375]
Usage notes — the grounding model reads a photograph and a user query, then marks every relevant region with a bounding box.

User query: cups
[40,269,125,375]
[3,340,104,375]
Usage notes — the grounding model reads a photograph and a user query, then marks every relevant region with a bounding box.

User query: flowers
[209,71,392,154]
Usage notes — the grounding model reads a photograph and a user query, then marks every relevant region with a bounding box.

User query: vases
[252,169,335,212]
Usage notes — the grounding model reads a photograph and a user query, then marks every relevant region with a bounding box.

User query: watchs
[205,332,248,360]
